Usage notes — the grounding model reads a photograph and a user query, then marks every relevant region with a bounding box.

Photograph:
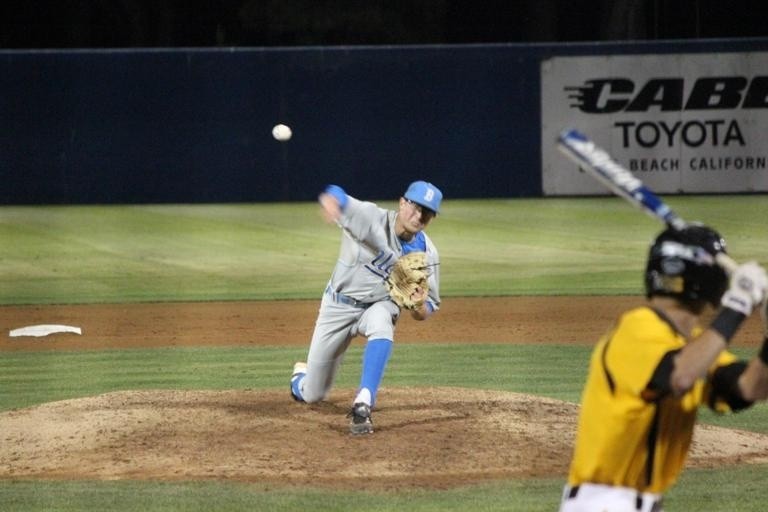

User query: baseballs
[272,124,292,141]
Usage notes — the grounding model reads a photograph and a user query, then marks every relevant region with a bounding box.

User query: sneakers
[349,402,373,434]
[291,362,306,402]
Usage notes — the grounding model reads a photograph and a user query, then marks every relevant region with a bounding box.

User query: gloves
[720,260,767,317]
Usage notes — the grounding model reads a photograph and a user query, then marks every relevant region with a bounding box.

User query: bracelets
[758,336,768,366]
[709,307,747,341]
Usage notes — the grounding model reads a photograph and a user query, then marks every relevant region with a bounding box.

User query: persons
[558,223,768,512]
[291,180,443,435]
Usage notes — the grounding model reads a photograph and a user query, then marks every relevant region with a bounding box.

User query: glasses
[404,198,435,214]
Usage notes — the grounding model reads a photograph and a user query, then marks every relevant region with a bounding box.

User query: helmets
[645,224,728,304]
[403,180,443,213]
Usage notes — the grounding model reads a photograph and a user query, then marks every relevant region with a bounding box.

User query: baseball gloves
[385,252,432,309]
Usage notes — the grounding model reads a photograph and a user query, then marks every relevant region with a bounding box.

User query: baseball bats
[553,127,768,304]
